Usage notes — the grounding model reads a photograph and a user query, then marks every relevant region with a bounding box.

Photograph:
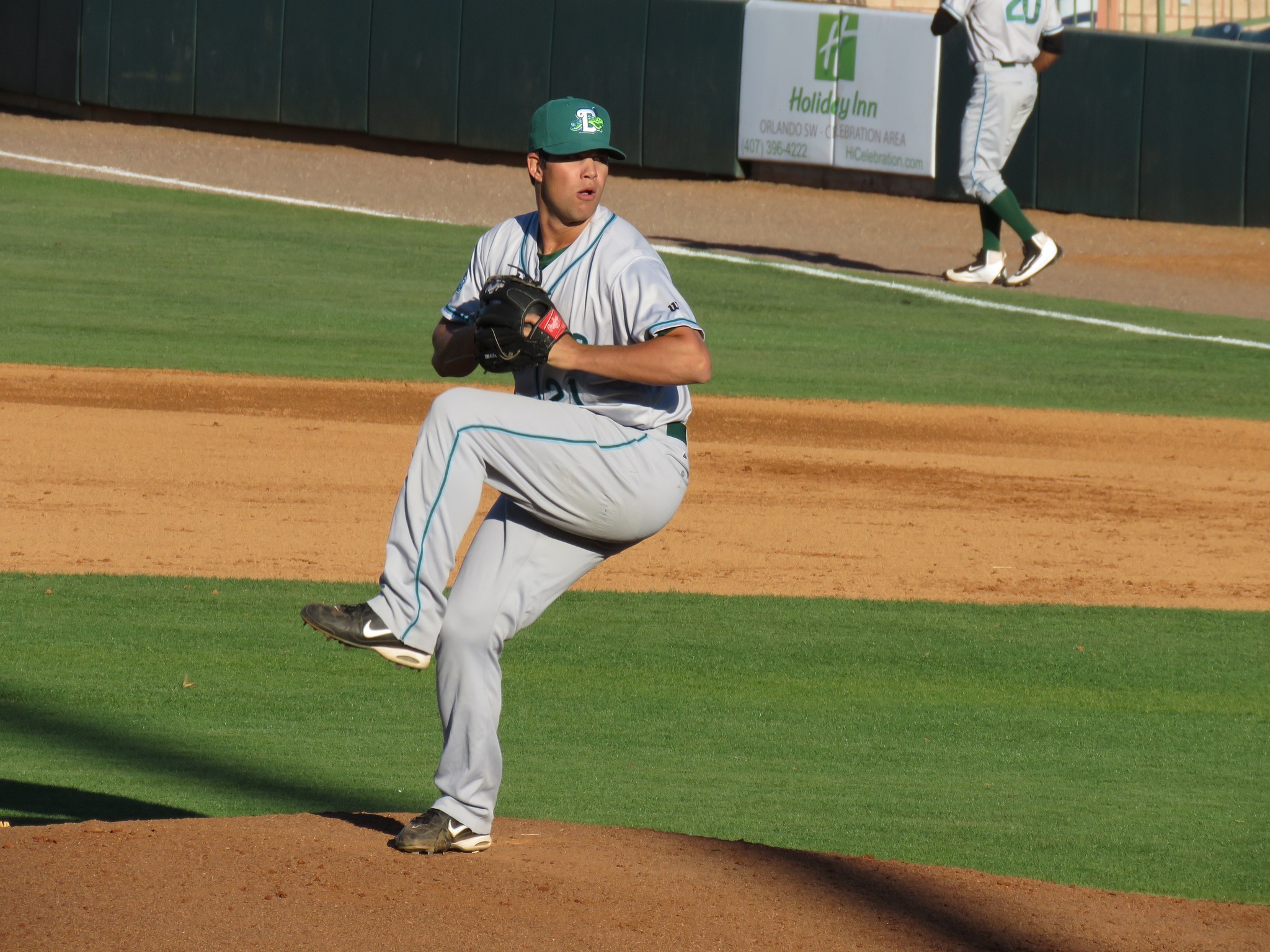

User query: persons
[302,100,711,852]
[931,0,1066,287]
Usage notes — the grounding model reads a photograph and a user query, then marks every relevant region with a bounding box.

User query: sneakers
[300,602,433,672]
[1004,231,1063,286]
[942,248,1008,284]
[394,808,492,854]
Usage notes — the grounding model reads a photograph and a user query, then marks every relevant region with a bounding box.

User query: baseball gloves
[473,262,574,376]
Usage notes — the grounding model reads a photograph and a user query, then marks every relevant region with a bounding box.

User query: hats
[528,96,626,161]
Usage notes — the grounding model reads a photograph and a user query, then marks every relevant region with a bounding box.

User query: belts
[666,422,687,446]
[1001,61,1016,68]
